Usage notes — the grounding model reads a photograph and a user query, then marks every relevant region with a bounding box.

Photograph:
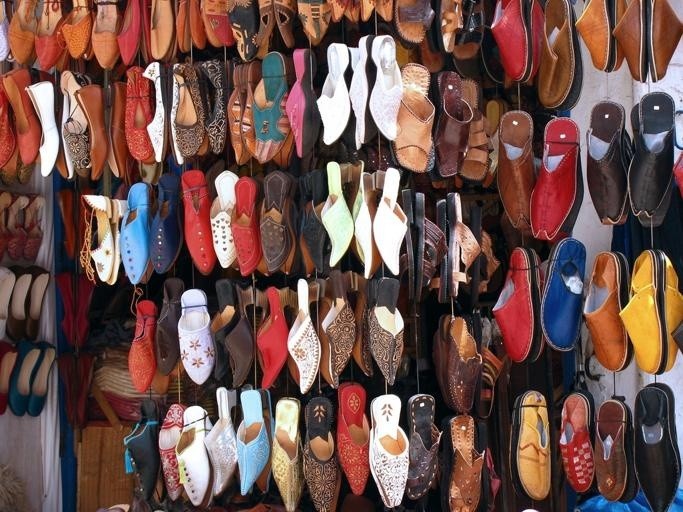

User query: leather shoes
[0,0,68,417]
